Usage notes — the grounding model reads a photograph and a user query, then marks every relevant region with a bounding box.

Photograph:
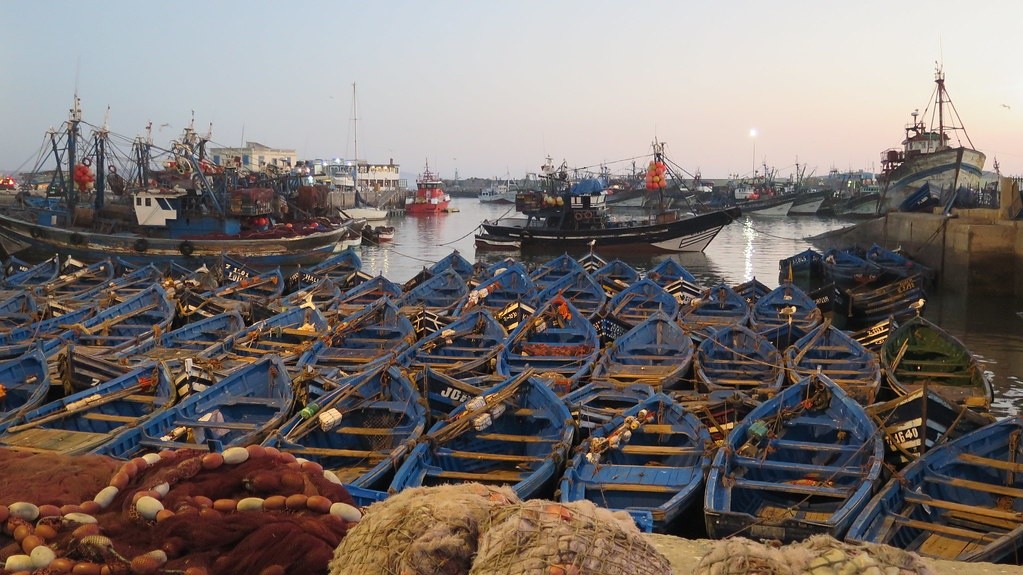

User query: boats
[873,59,988,214]
[477,166,522,204]
[87,349,298,466]
[0,79,355,274]
[404,158,451,213]
[1,356,176,458]
[0,337,52,433]
[556,386,716,534]
[703,364,886,543]
[259,354,429,490]
[385,363,576,503]
[843,414,1023,564]
[1,242,1023,474]
[332,216,397,254]
[472,136,884,257]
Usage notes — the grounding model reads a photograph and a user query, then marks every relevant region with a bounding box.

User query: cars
[0,178,15,190]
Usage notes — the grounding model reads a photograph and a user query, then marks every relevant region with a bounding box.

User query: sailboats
[339,82,388,222]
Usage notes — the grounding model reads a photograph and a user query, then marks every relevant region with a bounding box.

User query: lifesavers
[70,234,84,245]
[574,212,583,221]
[108,165,117,174]
[30,226,41,238]
[134,239,148,252]
[585,211,593,219]
[180,241,193,256]
[82,157,92,167]
[520,230,533,244]
